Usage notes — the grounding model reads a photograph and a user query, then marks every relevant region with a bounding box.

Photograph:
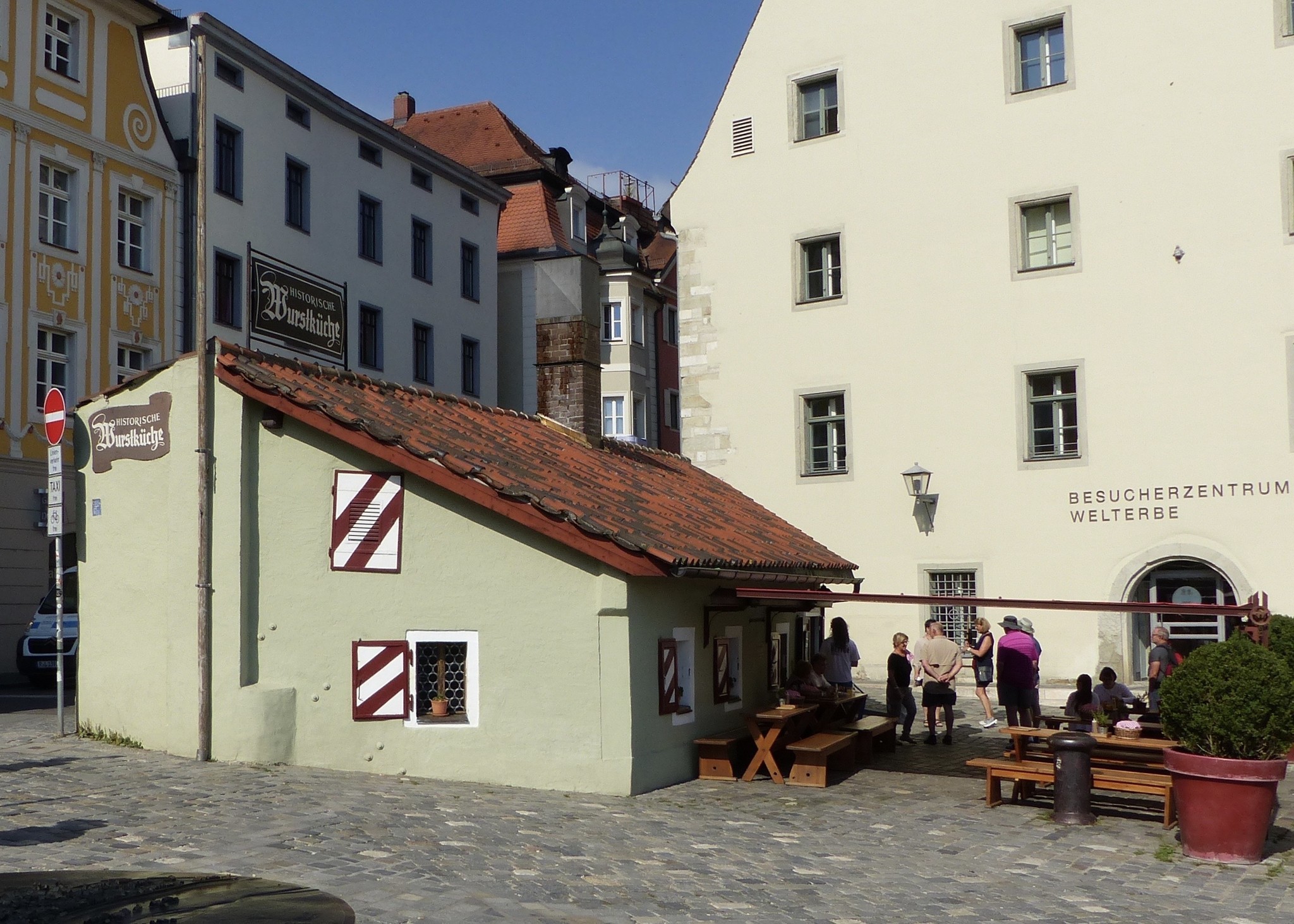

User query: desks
[1059,705,1161,723]
[807,690,870,730]
[1036,712,1163,736]
[740,702,820,782]
[998,725,1187,820]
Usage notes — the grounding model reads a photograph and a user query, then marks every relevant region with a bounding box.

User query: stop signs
[43,388,66,446]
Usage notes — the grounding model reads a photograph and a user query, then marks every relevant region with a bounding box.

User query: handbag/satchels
[978,666,993,683]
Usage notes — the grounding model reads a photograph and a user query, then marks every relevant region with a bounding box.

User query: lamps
[899,462,937,505]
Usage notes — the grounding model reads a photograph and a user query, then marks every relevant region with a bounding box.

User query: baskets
[1114,726,1143,740]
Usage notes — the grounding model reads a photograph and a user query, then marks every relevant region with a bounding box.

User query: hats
[1017,617,1035,633]
[998,616,1021,630]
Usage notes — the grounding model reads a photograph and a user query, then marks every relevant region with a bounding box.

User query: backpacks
[1156,644,1183,676]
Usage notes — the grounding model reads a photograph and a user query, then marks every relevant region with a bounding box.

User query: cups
[847,689,852,696]
[779,698,785,707]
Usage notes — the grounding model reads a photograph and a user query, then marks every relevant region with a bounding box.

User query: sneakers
[984,718,996,727]
[979,721,985,725]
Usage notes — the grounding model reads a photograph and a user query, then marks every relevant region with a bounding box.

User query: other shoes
[942,737,952,745]
[925,738,936,744]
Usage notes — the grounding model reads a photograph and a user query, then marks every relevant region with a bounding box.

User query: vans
[16,565,81,690]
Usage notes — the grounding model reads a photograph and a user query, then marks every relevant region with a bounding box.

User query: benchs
[842,714,900,765]
[691,723,770,782]
[966,739,1177,831]
[784,729,858,788]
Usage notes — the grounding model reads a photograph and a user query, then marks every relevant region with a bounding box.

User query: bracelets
[894,686,899,689]
[967,647,971,651]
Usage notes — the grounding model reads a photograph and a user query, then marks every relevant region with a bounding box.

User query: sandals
[923,720,929,727]
[935,719,943,726]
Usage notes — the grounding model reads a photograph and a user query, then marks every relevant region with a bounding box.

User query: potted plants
[431,695,449,714]
[1090,707,1112,736]
[1155,608,1294,866]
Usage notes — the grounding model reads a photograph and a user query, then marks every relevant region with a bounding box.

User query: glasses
[1152,634,1159,637]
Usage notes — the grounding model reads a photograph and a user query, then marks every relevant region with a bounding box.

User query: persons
[1093,667,1137,733]
[886,632,917,744]
[1065,674,1100,732]
[996,615,1039,750]
[822,617,860,697]
[919,622,963,746]
[1016,618,1042,743]
[784,653,838,704]
[912,618,944,727]
[962,617,998,728]
[1148,627,1173,711]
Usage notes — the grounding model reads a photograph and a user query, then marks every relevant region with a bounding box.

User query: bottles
[852,683,855,695]
[1092,718,1097,733]
[834,684,839,697]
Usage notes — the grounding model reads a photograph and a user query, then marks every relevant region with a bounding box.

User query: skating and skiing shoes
[899,735,917,745]
